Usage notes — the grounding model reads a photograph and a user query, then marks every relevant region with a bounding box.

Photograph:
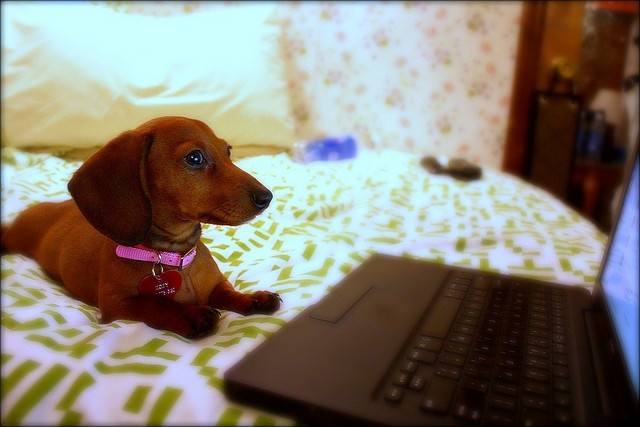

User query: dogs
[0,115,284,339]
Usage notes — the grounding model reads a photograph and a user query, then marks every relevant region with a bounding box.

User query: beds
[1,0,607,427]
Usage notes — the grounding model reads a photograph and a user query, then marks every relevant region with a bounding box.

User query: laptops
[222,137,640,425]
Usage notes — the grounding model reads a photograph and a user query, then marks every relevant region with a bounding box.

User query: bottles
[291,130,374,165]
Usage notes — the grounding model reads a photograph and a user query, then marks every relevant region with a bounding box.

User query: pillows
[2,1,295,149]
